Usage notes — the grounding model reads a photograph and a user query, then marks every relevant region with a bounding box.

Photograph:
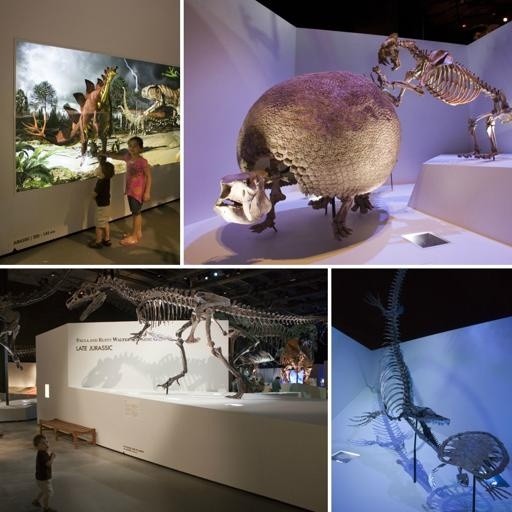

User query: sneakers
[32,499,56,512]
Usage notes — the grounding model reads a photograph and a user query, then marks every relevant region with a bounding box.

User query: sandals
[124,233,141,241]
[88,239,105,249]
[101,238,113,248]
[120,236,138,246]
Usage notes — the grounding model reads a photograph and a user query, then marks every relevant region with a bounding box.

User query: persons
[93,137,153,247]
[230,376,266,393]
[272,377,283,393]
[32,435,58,512]
[88,138,116,250]
